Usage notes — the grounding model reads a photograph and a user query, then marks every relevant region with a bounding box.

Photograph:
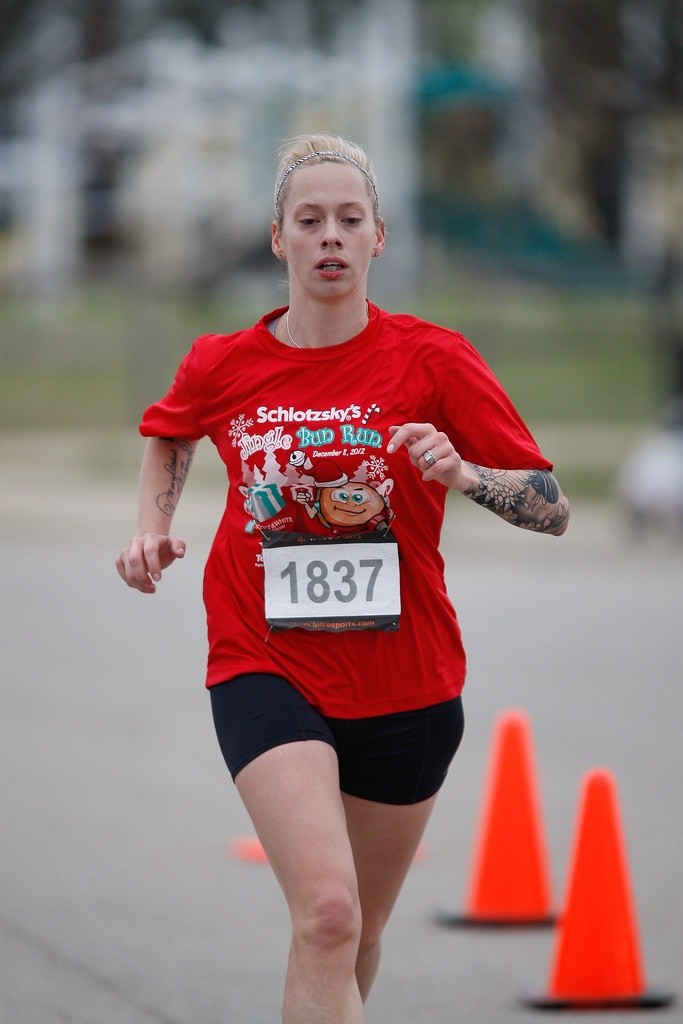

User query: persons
[115,126,568,1024]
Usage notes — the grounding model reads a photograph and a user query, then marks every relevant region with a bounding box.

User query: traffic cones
[438,710,564,929]
[525,773,677,1009]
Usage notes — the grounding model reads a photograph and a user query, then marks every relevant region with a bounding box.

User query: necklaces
[286,309,303,350]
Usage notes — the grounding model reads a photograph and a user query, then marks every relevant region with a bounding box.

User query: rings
[421,451,437,468]
